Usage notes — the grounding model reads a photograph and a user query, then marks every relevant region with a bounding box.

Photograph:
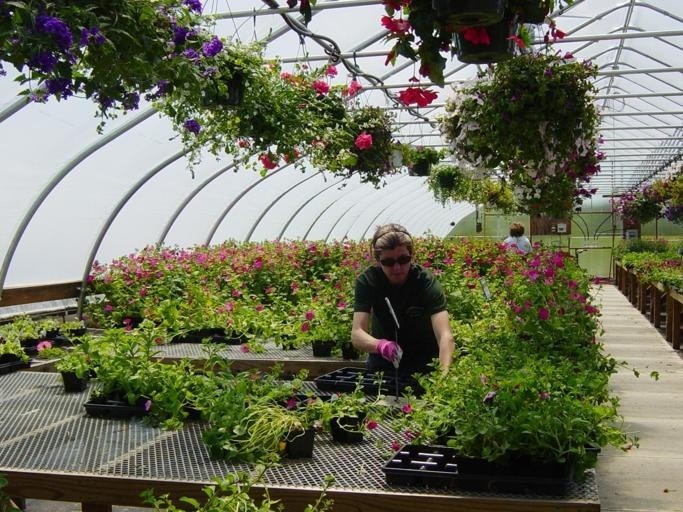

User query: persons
[351,223,455,395]
[502,223,532,253]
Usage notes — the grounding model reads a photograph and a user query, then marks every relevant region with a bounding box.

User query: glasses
[381,256,411,266]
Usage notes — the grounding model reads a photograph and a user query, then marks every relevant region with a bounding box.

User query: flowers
[0,0,680,222]
[34,237,618,454]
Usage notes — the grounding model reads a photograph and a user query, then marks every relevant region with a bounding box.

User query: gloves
[377,339,403,368]
